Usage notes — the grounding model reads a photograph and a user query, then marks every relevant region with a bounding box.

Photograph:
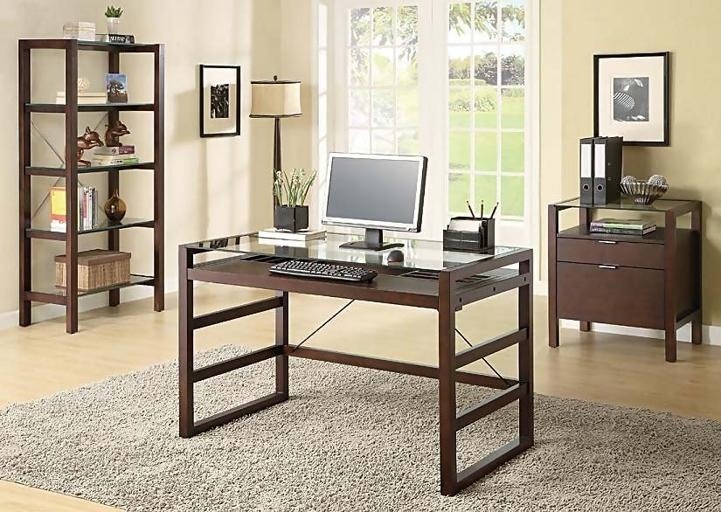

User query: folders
[579,136,607,205]
[594,137,622,204]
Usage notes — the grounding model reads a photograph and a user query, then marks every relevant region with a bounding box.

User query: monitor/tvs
[321,152,428,252]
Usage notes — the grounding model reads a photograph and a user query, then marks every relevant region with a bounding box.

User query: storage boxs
[53,249,131,293]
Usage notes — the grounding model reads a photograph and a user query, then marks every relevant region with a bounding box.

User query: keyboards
[268,260,377,281]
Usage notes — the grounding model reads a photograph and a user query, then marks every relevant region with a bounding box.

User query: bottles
[104,188,127,226]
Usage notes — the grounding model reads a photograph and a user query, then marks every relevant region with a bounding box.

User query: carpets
[0,345,721,511]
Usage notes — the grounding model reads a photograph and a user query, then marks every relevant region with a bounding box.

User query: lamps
[248,74,300,227]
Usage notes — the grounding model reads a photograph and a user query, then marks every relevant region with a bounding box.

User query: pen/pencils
[480,200,484,219]
[490,202,499,219]
[467,201,475,218]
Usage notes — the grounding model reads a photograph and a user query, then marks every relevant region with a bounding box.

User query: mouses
[388,250,403,262]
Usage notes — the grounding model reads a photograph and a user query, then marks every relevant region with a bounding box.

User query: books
[258,228,328,241]
[56,92,106,104]
[259,238,326,248]
[90,146,139,166]
[50,186,99,233]
[95,34,135,44]
[590,218,657,236]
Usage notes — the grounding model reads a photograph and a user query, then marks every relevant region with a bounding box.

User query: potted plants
[269,166,316,230]
[104,6,123,34]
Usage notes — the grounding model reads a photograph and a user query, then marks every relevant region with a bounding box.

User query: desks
[179,229,532,496]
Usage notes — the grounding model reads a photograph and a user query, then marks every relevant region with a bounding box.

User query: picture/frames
[593,51,670,146]
[199,64,241,138]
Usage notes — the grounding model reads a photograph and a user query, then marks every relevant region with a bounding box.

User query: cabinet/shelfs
[547,195,701,361]
[18,33,164,335]
